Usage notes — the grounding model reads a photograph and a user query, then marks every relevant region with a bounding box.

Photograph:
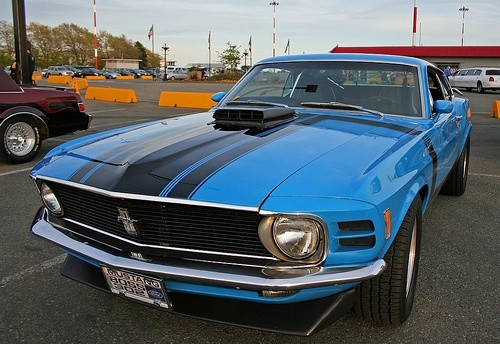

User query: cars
[0,65,93,165]
[447,67,500,94]
[41,64,214,81]
[4,66,12,75]
[28,52,474,338]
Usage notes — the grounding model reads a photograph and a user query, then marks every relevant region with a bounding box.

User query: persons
[381,71,396,80]
[10,62,16,79]
[444,66,452,76]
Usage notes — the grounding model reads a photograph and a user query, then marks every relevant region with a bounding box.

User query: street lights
[243,52,248,73]
[458,5,469,46]
[162,44,170,81]
[268,1,280,57]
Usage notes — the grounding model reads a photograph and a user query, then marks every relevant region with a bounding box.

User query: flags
[148,26,153,39]
[284,41,289,53]
